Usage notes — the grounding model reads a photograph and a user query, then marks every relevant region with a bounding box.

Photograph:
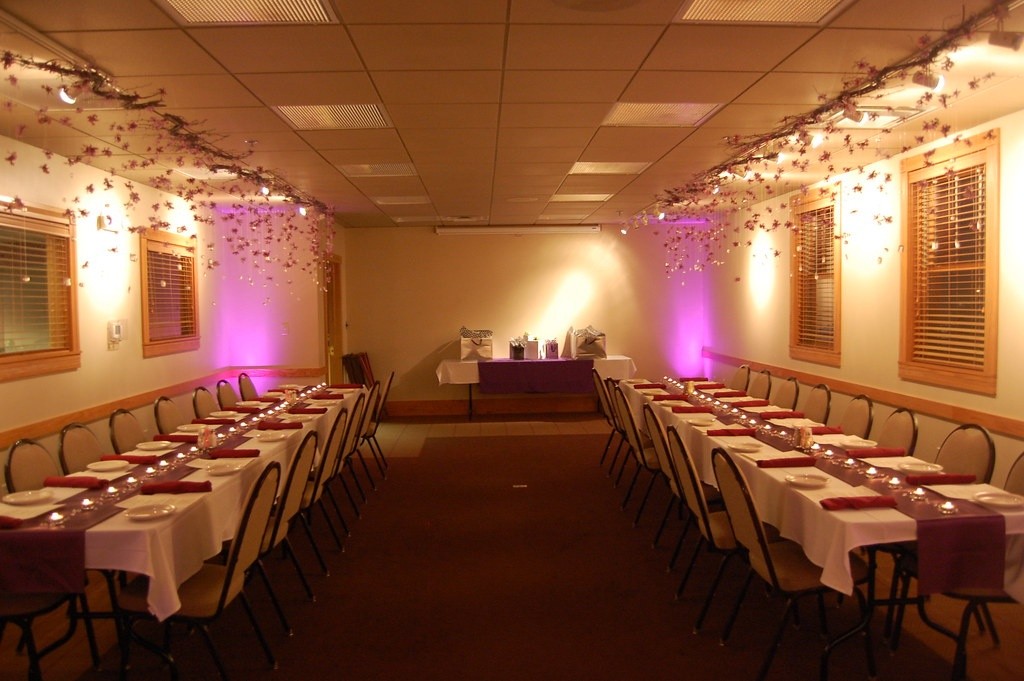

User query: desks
[436,355,638,422]
[618,379,1024,681]
[0,384,369,680]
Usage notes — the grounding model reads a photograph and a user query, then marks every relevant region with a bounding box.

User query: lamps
[434,224,603,235]
[989,2,1024,50]
[732,133,813,178]
[913,62,941,90]
[57,78,86,105]
[843,108,864,122]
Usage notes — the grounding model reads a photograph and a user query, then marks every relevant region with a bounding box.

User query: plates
[973,492,1024,507]
[333,389,354,393]
[255,432,285,442]
[205,463,242,475]
[625,379,646,383]
[210,411,237,417]
[641,390,665,395]
[659,401,686,407]
[313,400,337,406]
[279,384,298,388]
[176,424,206,432]
[263,392,283,397]
[784,474,830,488]
[2,490,53,504]
[839,438,877,449]
[136,441,171,451]
[87,460,129,471]
[286,415,313,421]
[898,462,943,475]
[728,442,764,452]
[686,418,716,426]
[125,503,176,520]
[235,401,261,407]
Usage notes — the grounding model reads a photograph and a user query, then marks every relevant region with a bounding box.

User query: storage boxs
[510,340,538,359]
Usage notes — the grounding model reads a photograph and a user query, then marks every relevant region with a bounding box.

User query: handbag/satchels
[461,330,494,360]
[571,333,607,360]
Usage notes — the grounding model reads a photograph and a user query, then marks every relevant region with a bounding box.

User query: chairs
[590,365,1024,680]
[0,373,394,681]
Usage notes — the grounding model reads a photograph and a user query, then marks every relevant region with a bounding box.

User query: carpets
[76,435,955,681]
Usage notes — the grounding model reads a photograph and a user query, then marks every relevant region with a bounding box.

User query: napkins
[140,481,212,493]
[756,457,817,468]
[671,406,713,413]
[706,429,756,436]
[713,391,746,397]
[760,411,805,420]
[288,408,328,414]
[101,456,157,463]
[634,384,666,389]
[311,393,343,399]
[0,515,21,527]
[155,434,198,443]
[820,496,896,510]
[731,400,769,408]
[679,377,709,382]
[223,406,261,414]
[695,383,725,389]
[907,473,977,485]
[809,426,845,435]
[257,420,303,430]
[207,449,261,458]
[268,388,299,393]
[653,394,688,401]
[846,447,907,458]
[330,383,364,388]
[191,418,236,424]
[248,397,280,403]
[45,476,110,490]
